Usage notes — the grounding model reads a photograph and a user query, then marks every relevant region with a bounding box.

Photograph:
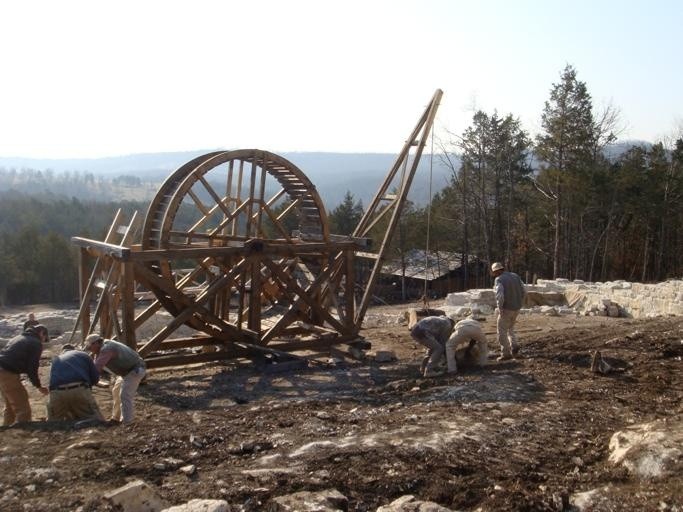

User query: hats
[82,334,100,351]
[492,262,504,272]
[38,324,50,342]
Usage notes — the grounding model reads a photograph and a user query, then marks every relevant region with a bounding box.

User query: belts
[50,383,84,390]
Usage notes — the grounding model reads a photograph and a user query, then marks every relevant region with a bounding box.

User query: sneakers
[496,356,515,363]
[419,365,445,378]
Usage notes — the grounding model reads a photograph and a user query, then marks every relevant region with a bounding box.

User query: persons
[23,313,38,332]
[444,319,487,373]
[410,315,456,377]
[0,324,50,427]
[82,334,145,422]
[491,262,526,362]
[45,343,105,422]
